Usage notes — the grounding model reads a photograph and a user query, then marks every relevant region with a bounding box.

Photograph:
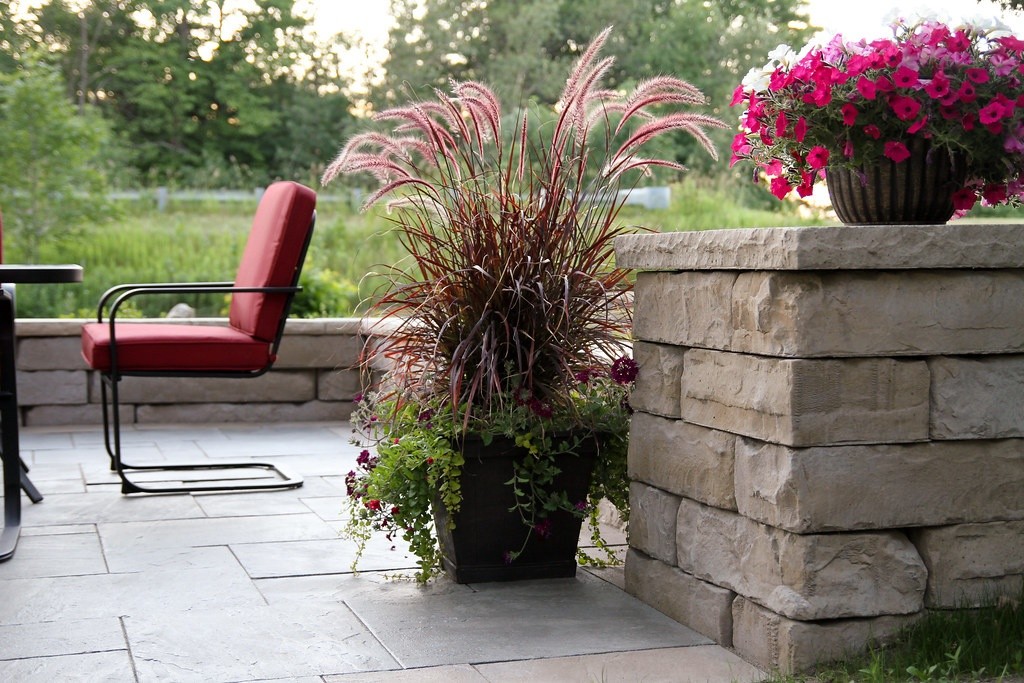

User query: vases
[826,151,961,225]
[430,490,588,583]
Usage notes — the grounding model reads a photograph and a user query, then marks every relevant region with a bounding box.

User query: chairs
[80,180,318,493]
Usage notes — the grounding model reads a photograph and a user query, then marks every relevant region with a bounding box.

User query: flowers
[729,4,1024,226]
[319,23,732,586]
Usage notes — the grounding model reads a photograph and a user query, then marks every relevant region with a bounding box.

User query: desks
[0,264,81,502]
